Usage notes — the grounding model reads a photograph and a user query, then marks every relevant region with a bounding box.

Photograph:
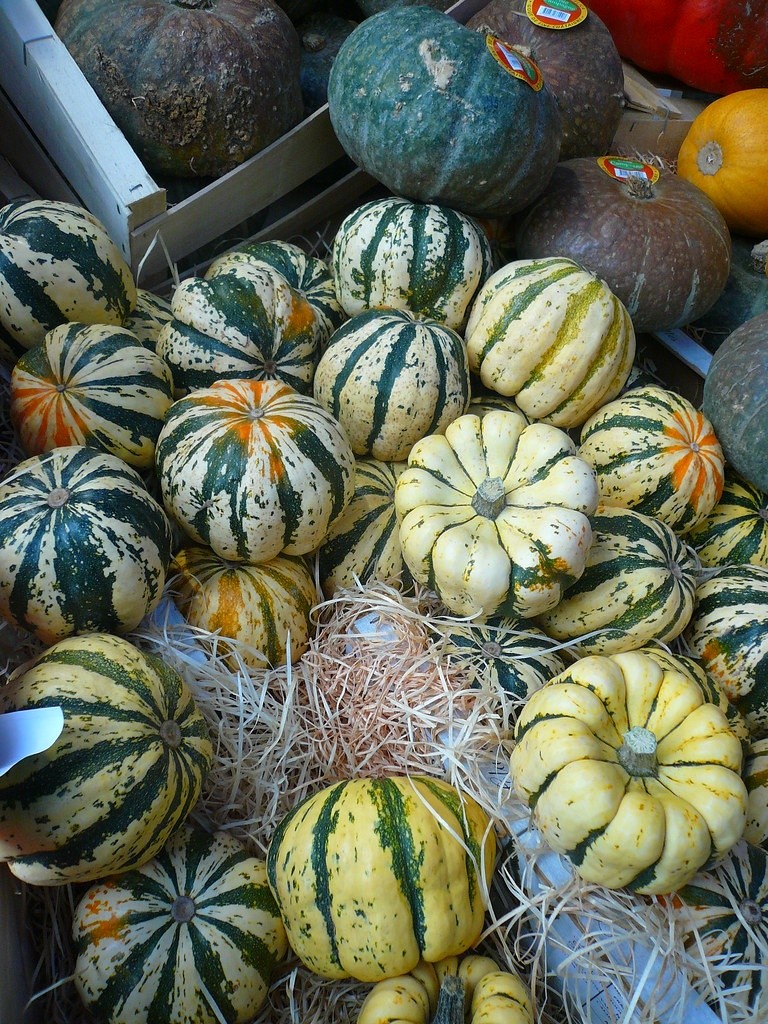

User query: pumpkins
[0,0,768,1024]
